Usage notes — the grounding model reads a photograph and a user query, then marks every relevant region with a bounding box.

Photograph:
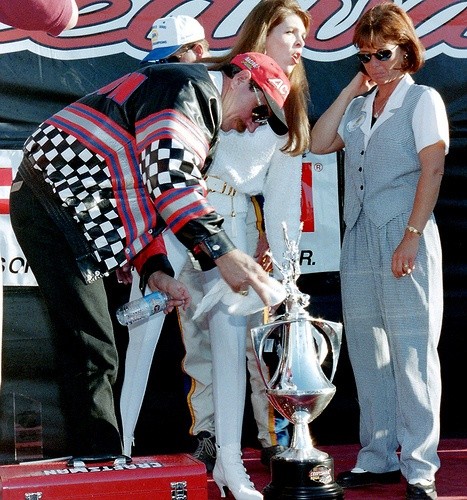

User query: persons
[8,0,312,500]
[308,4,450,500]
[0,0,78,38]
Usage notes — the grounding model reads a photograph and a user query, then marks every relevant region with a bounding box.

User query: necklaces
[372,90,389,119]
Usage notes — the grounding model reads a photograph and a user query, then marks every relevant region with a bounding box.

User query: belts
[203,175,244,198]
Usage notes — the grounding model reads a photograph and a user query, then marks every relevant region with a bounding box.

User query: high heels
[121,433,136,463]
[213,443,264,500]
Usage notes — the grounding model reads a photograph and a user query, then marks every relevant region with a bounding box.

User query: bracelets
[406,224,424,236]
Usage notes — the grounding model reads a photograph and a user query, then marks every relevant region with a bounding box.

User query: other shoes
[190,430,217,473]
[406,481,438,500]
[261,445,288,466]
[336,469,402,488]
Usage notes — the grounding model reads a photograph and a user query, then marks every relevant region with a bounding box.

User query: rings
[403,263,409,268]
[239,289,250,295]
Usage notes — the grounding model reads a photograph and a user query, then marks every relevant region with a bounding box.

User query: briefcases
[0,452,210,499]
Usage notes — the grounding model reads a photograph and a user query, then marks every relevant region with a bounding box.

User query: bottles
[116,290,173,326]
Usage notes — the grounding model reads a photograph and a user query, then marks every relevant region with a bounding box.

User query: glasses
[354,44,398,64]
[159,44,197,64]
[251,85,269,126]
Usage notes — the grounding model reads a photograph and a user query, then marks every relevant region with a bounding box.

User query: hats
[140,15,205,64]
[230,52,291,136]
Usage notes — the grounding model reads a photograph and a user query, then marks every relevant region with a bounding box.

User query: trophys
[250,221,343,500]
